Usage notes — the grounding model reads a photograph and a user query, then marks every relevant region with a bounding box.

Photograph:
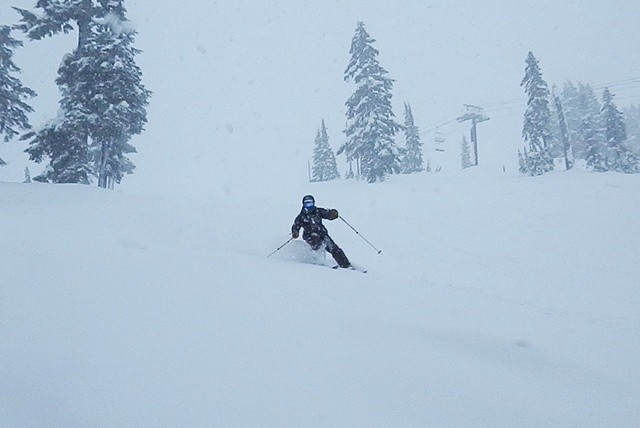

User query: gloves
[330,209,339,219]
[291,230,299,239]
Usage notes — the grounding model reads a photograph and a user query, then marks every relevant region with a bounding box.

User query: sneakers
[342,263,353,270]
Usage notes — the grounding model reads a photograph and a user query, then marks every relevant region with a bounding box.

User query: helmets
[303,195,315,209]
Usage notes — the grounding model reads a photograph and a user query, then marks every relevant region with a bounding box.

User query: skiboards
[310,262,367,273]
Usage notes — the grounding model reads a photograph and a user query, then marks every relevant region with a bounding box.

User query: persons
[292,195,355,270]
